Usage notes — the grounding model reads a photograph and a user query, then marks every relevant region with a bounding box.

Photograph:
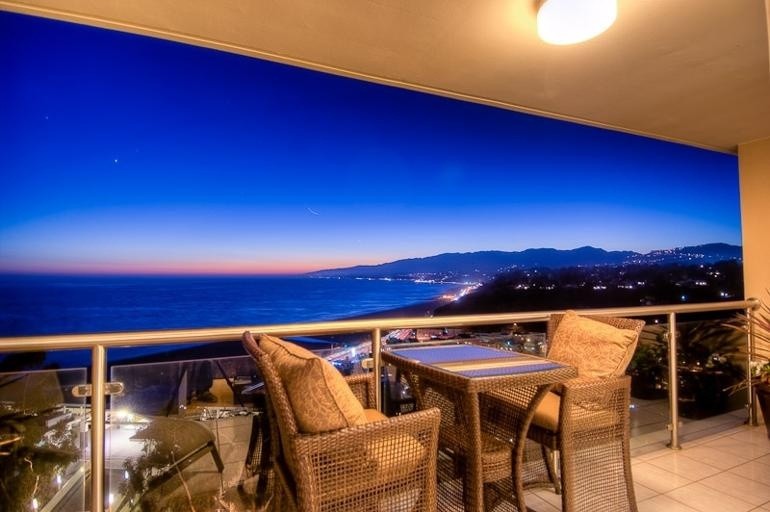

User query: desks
[375,339,581,511]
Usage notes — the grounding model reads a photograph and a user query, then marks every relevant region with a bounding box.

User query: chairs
[235,328,445,512]
[0,368,229,511]
[488,309,646,512]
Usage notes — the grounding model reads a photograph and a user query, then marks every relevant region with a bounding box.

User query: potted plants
[636,315,745,423]
[714,285,770,443]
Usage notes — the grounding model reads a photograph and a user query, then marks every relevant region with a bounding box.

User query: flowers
[703,350,727,370]
[747,356,769,377]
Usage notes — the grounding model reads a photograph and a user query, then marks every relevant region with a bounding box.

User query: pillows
[539,308,642,417]
[258,332,384,474]
